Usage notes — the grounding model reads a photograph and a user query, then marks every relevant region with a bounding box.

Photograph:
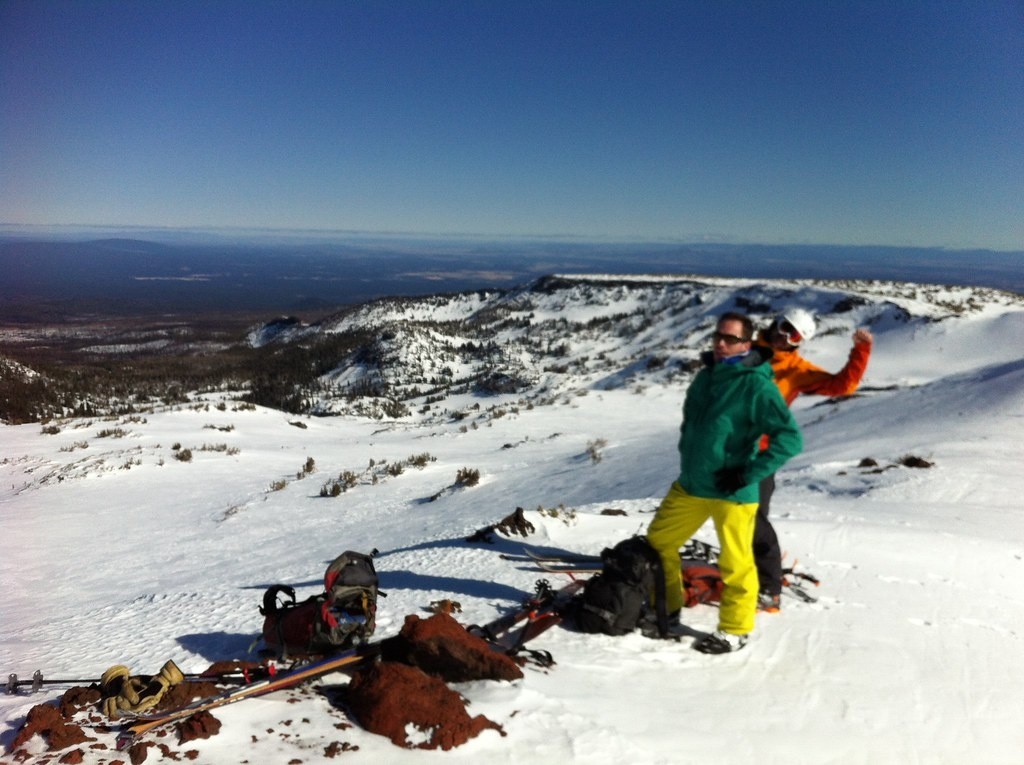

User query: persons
[641,310,805,654]
[753,309,872,612]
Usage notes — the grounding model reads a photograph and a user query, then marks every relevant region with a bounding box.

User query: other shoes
[635,609,681,629]
[695,630,749,654]
[756,589,781,612]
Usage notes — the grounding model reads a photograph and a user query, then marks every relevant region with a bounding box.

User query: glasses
[774,317,805,346]
[711,330,751,344]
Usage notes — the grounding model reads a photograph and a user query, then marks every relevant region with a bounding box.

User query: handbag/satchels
[257,550,387,660]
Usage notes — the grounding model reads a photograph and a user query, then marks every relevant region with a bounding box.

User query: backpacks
[677,558,725,608]
[558,535,680,642]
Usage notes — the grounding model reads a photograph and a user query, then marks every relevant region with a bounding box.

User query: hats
[783,308,816,341]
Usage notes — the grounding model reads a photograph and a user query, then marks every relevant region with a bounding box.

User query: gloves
[101,664,140,718]
[123,659,183,713]
[714,465,746,497]
